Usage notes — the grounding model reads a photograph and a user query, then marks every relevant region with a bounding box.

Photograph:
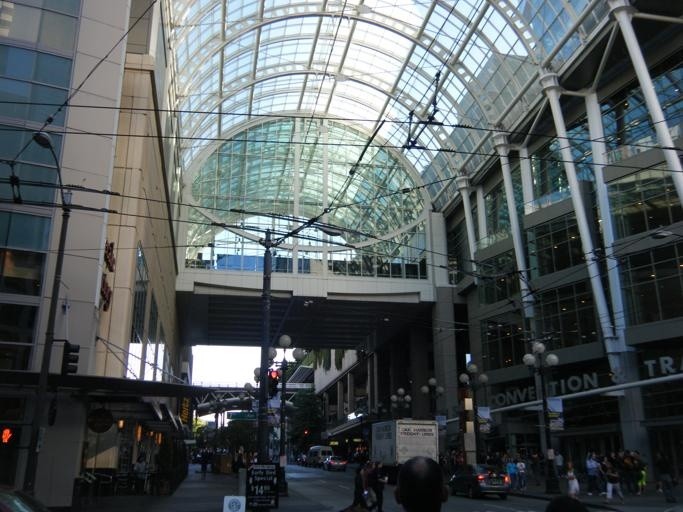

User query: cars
[323,455,348,471]
[447,463,510,499]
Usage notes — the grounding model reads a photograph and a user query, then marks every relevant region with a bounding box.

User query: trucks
[371,421,441,483]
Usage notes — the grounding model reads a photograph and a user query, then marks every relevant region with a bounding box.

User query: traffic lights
[60,340,81,385]
[268,372,279,397]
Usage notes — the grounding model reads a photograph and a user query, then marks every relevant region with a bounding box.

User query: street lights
[654,230,683,242]
[458,364,488,464]
[420,378,444,420]
[451,269,548,454]
[244,368,260,403]
[270,335,306,497]
[372,388,412,419]
[24,130,70,494]
[523,342,561,494]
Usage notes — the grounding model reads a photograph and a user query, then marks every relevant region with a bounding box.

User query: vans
[306,446,334,468]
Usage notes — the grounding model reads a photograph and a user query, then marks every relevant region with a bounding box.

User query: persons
[132,455,147,496]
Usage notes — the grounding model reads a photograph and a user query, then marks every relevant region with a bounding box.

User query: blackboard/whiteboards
[246,463,280,510]
